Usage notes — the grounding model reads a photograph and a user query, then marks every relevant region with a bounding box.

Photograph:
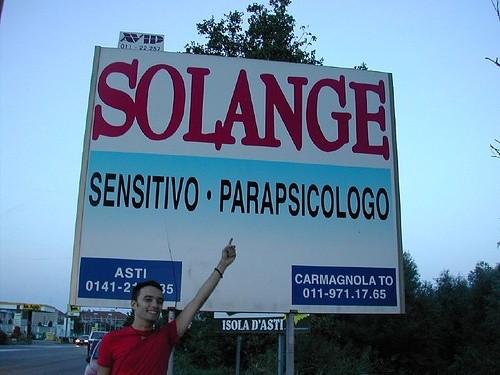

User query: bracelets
[215,267,224,279]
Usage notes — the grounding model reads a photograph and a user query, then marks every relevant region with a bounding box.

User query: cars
[75,334,90,345]
[85,331,113,375]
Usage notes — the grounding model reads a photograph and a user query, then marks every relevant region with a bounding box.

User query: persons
[96,237,236,375]
